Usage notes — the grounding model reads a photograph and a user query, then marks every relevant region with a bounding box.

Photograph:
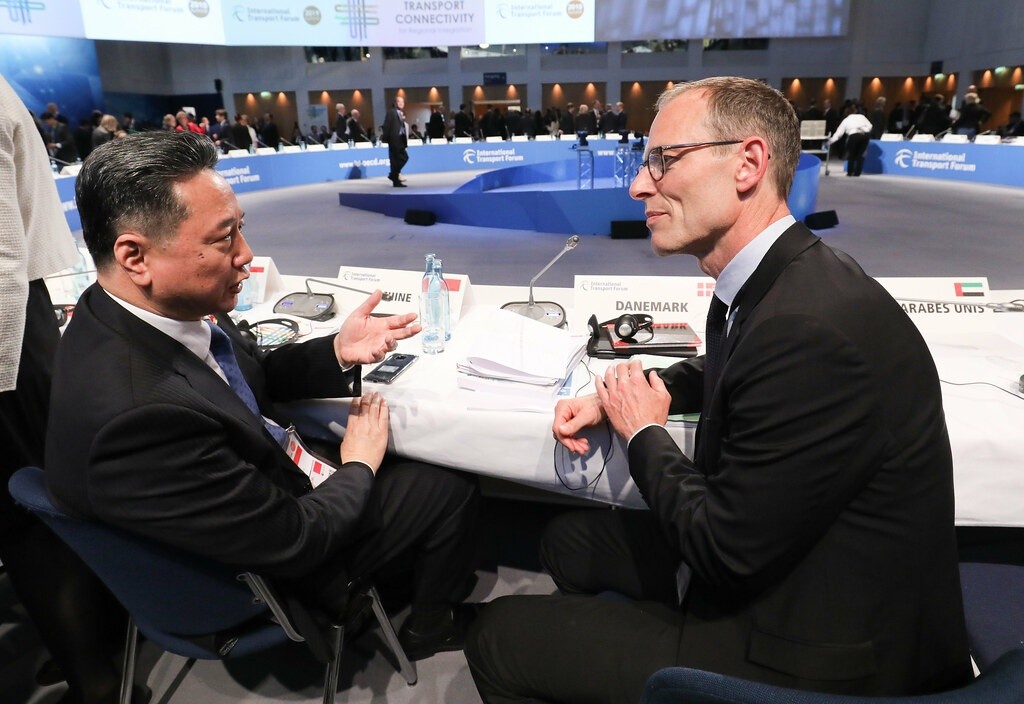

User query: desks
[237,275,1024,528]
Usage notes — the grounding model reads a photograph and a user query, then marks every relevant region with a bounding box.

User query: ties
[705,292,729,414]
[205,319,288,448]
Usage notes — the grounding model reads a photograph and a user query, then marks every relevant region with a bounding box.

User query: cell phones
[361,352,420,384]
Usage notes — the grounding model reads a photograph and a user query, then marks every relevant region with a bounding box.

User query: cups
[419,291,445,354]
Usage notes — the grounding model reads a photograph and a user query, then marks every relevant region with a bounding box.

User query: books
[456,308,590,387]
[629,355,700,428]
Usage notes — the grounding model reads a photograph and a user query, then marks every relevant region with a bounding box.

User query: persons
[827,105,873,176]
[29,102,279,172]
[0,73,151,704]
[291,97,627,187]
[463,76,972,704]
[43,130,483,659]
[788,85,1024,160]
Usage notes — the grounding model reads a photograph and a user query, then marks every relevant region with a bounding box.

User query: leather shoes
[398,600,478,662]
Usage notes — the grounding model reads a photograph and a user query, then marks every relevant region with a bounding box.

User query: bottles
[428,259,451,341]
[234,263,252,311]
[422,253,436,292]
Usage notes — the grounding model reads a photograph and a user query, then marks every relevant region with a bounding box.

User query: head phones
[54,302,77,326]
[235,318,300,350]
[587,314,654,339]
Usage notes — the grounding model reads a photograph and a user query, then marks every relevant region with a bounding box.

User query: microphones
[904,124,991,142]
[222,123,511,150]
[273,278,394,322]
[500,234,581,328]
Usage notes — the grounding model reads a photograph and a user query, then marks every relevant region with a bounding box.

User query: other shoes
[387,175,408,188]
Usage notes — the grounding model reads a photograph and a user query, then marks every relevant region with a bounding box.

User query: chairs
[8,465,418,704]
[644,565,1024,704]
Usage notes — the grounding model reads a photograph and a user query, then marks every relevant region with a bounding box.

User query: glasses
[638,140,770,181]
